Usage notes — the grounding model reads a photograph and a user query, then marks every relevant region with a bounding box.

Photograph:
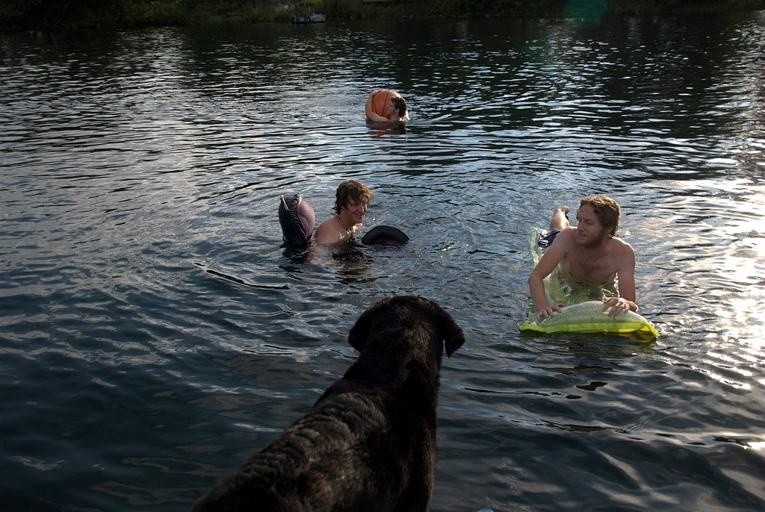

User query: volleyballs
[372,88,399,120]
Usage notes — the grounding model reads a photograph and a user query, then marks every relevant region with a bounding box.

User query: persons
[307,181,371,272]
[528,194,638,321]
[364,88,410,125]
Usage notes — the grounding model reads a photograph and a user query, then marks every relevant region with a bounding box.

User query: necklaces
[335,214,354,231]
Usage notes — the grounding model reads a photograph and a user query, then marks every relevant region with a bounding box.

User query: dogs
[191,294,466,512]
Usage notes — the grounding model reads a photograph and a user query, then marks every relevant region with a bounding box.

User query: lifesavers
[519,226,660,338]
